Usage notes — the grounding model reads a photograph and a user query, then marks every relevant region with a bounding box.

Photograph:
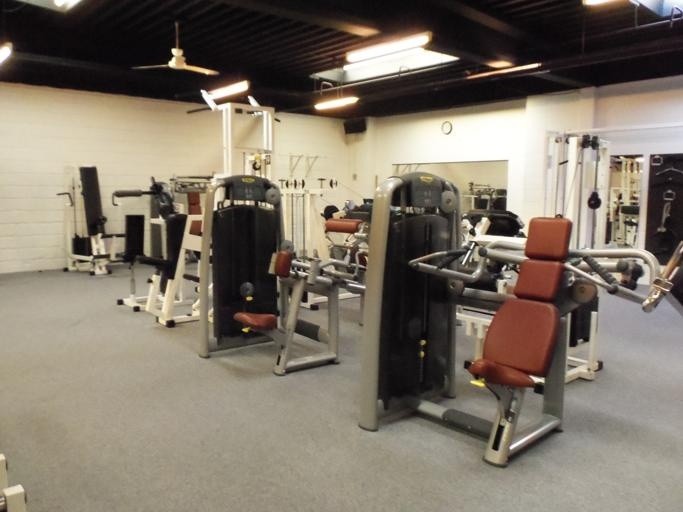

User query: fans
[128,21,223,78]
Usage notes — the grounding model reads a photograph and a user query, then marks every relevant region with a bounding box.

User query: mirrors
[390,160,508,219]
[604,153,644,248]
